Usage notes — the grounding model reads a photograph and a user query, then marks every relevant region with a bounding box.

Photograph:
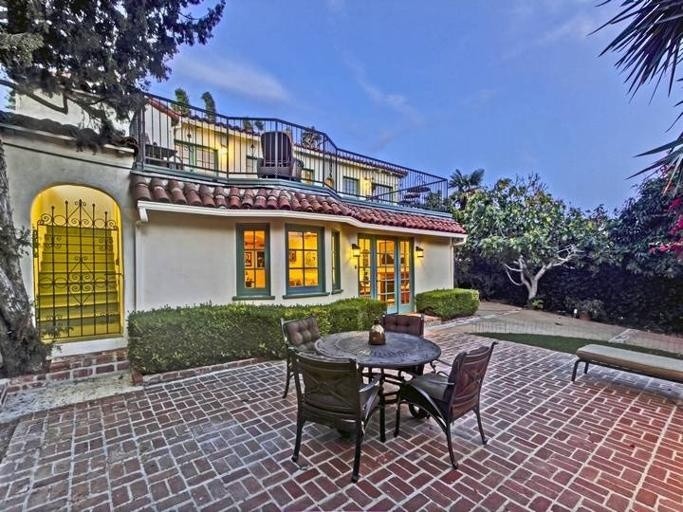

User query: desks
[317,331,441,443]
[147,143,176,166]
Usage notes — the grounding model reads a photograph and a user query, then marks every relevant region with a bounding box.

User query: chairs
[278,317,321,398]
[287,347,386,483]
[383,313,424,377]
[403,187,430,204]
[394,341,499,470]
[257,132,303,180]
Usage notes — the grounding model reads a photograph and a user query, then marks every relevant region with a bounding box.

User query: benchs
[571,344,683,385]
